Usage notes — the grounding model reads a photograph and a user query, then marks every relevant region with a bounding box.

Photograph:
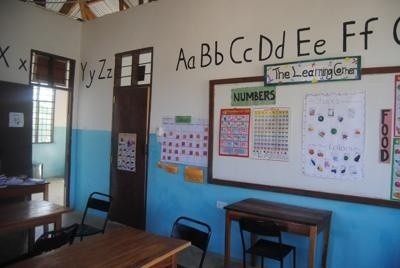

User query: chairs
[238,218,297,268]
[72,192,116,240]
[28,223,75,257]
[168,216,212,268]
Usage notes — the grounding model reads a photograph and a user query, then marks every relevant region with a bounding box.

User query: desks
[3,219,193,268]
[0,198,74,250]
[223,198,332,268]
[1,177,51,200]
